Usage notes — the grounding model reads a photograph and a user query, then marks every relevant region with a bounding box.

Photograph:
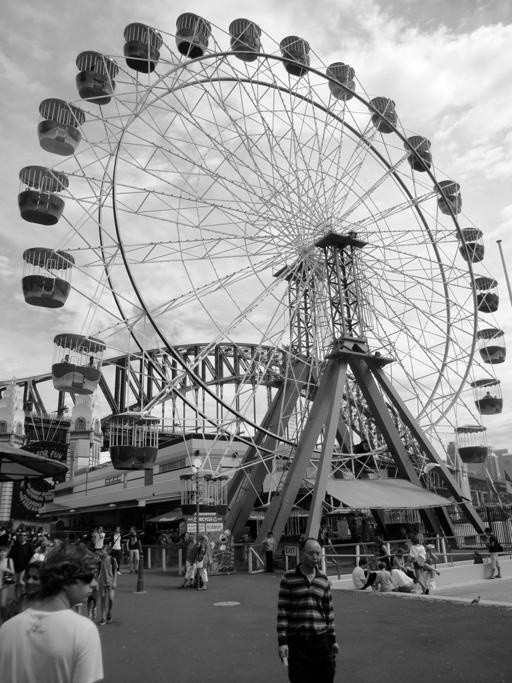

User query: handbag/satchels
[4,572,16,584]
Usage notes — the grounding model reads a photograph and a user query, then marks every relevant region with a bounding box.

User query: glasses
[68,570,95,584]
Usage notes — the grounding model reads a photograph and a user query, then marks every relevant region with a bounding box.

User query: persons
[0,523,143,626]
[276,537,340,682]
[84,355,98,370]
[351,532,443,595]
[178,532,209,590]
[0,540,106,682]
[262,530,276,572]
[61,353,73,364]
[484,526,503,579]
[483,391,494,399]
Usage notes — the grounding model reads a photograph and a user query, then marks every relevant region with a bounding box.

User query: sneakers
[108,613,112,621]
[99,619,106,626]
